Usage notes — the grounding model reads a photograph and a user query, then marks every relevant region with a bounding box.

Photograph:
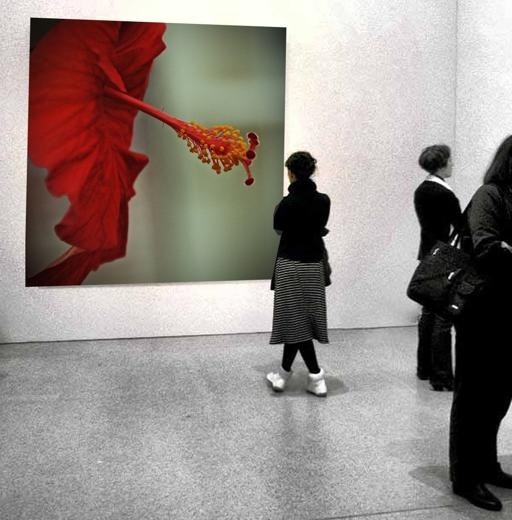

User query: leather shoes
[452,479,503,513]
[413,369,456,392]
[482,459,512,491]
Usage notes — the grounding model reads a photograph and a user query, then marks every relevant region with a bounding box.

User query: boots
[304,367,328,397]
[266,364,294,393]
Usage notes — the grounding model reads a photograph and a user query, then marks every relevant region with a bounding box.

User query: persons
[263,150,337,397]
[413,140,463,392]
[448,134,512,514]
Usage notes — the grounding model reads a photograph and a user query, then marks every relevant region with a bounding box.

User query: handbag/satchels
[406,200,484,324]
